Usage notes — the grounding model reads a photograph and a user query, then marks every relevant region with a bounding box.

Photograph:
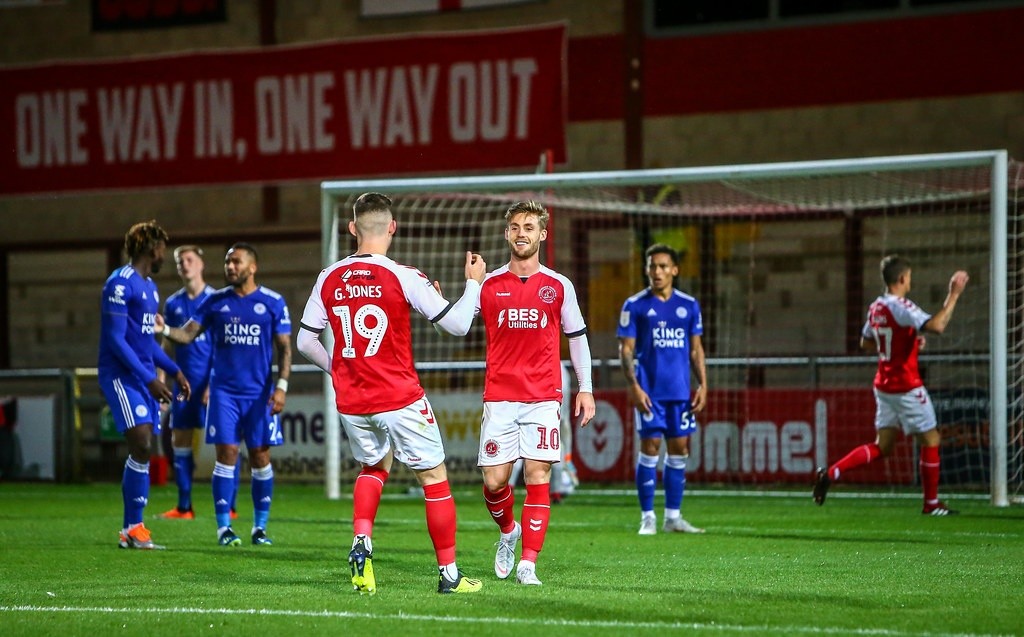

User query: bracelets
[276,378,288,392]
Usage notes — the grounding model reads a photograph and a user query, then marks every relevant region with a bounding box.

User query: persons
[813,254,969,517]
[296,192,486,595]
[97,220,291,550]
[615,243,707,535]
[433,200,596,586]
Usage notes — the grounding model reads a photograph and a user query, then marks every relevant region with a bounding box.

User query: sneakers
[118,525,166,550]
[813,467,830,506]
[348,539,376,597]
[663,516,705,533]
[219,530,241,548]
[638,515,656,534]
[438,568,482,593]
[517,565,542,586]
[922,502,960,516]
[250,526,272,544]
[493,521,522,579]
[230,510,238,518]
[161,508,193,519]
